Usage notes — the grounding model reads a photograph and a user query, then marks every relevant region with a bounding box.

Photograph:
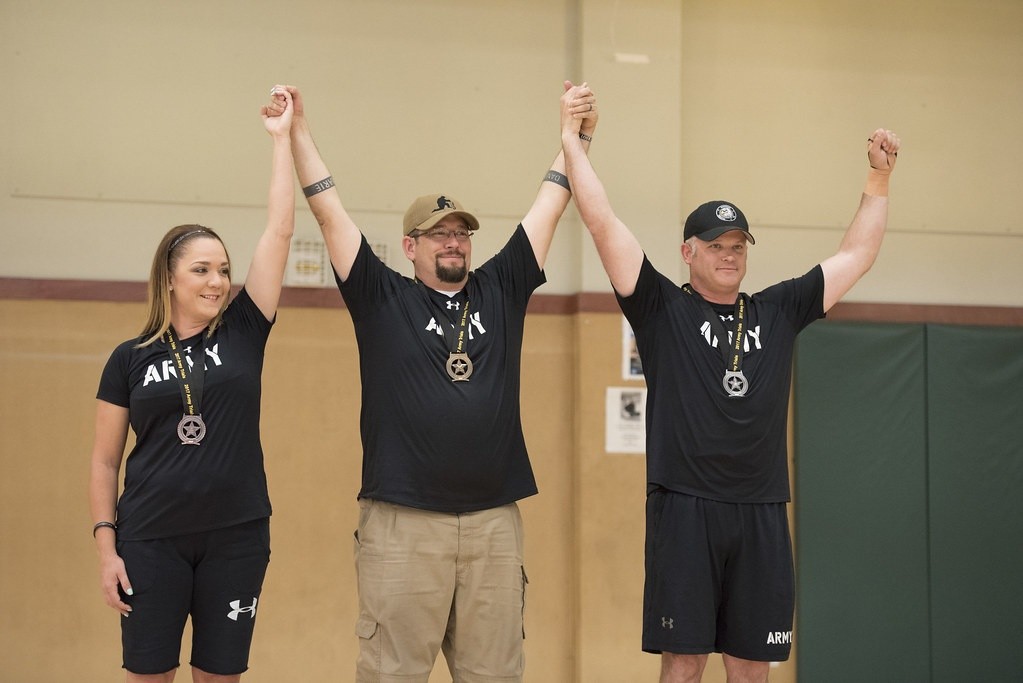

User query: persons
[560,82,901,683]
[90,87,295,683]
[266,78,599,683]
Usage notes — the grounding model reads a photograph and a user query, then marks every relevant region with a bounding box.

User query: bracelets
[579,131,592,141]
[93,522,116,538]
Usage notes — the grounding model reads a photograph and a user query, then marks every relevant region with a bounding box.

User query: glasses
[415,227,474,241]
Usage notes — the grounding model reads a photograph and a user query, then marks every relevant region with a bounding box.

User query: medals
[178,414,206,445]
[447,352,473,382]
[723,370,749,397]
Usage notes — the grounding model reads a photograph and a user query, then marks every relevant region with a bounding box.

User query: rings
[588,103,592,111]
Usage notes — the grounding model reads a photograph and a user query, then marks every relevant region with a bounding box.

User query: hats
[403,194,479,237]
[683,201,755,245]
